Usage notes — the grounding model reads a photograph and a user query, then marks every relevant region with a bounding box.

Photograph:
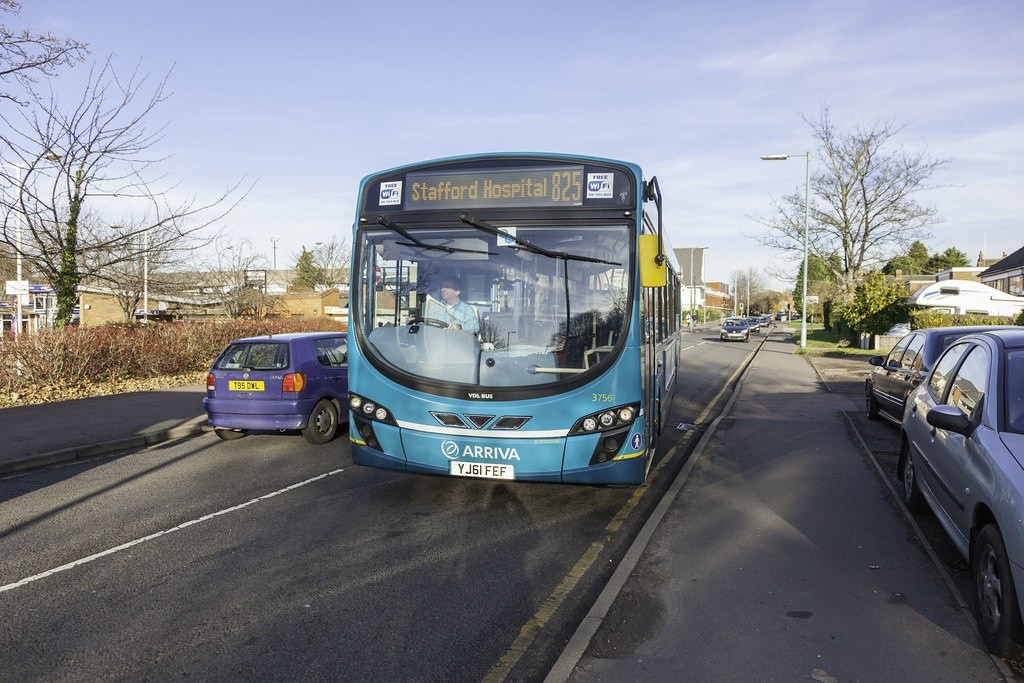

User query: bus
[344,152,683,487]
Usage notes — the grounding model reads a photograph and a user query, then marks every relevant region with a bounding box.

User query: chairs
[325,350,344,367]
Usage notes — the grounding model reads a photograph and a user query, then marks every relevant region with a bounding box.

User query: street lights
[111,224,148,323]
[14,152,64,333]
[690,246,710,333]
[760,150,810,349]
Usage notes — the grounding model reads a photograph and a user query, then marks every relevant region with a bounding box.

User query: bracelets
[457,324,462,330]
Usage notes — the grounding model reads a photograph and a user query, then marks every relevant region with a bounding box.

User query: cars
[719,318,751,343]
[864,325,1024,430]
[897,330,1024,657]
[203,331,348,444]
[748,311,784,333]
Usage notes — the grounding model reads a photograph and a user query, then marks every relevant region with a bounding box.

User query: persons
[685,313,691,326]
[692,313,697,326]
[418,275,480,334]
